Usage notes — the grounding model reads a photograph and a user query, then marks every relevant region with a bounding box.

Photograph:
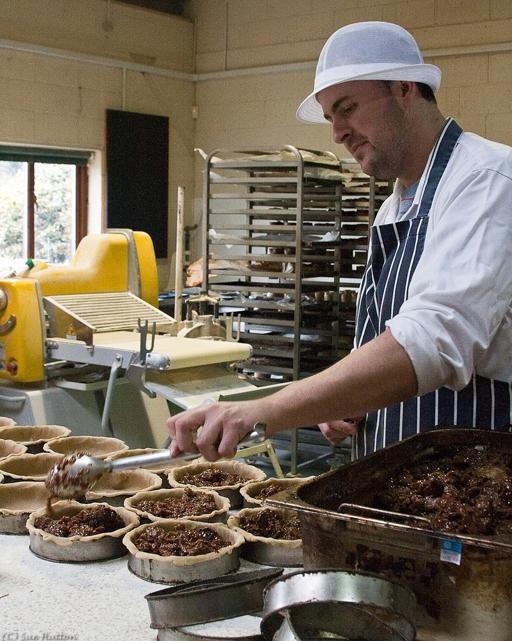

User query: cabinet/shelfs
[199,143,343,474]
[267,157,395,448]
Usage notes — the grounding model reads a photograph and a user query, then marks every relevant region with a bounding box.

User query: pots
[265,428,510,638]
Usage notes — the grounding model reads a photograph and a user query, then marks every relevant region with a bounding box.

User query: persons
[165,21,512,463]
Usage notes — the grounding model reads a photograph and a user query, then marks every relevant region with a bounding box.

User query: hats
[293,20,442,126]
